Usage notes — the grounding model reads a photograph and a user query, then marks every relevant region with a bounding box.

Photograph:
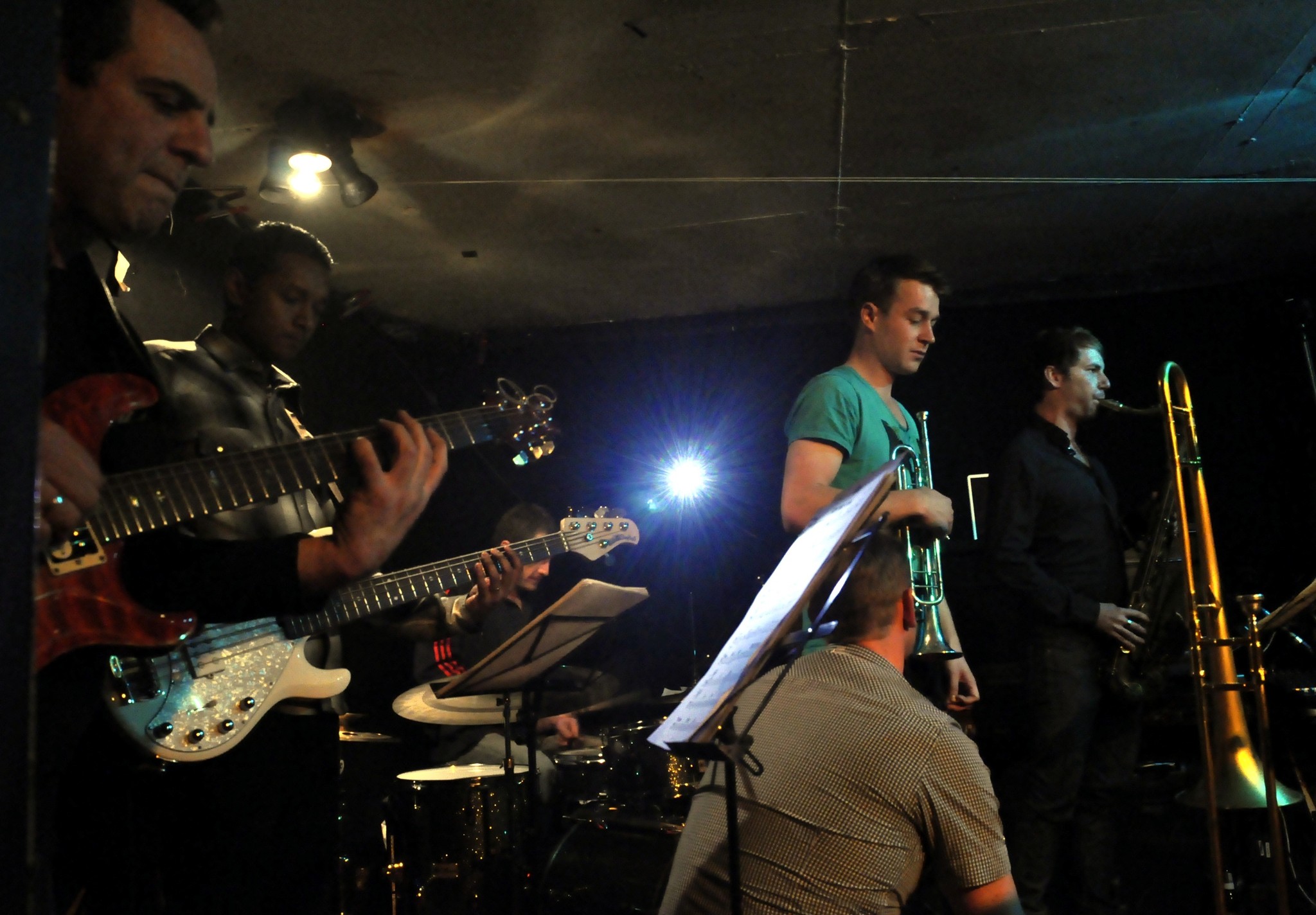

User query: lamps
[254,126,379,212]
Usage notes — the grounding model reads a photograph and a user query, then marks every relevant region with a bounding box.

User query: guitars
[87,497,652,761]
[30,362,561,676]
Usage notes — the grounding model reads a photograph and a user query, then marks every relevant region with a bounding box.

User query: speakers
[966,471,989,542]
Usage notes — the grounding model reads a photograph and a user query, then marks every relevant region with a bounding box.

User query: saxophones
[1093,393,1195,708]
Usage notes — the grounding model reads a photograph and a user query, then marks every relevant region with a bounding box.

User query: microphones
[1261,608,1312,656]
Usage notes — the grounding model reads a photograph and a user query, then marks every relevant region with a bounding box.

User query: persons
[775,255,982,726]
[657,523,1028,914]
[92,218,531,901]
[987,325,1154,915]
[410,499,621,843]
[0,0,453,915]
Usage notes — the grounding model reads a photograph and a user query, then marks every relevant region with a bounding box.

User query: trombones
[1154,356,1304,915]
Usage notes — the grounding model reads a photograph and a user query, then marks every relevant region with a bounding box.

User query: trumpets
[884,408,965,666]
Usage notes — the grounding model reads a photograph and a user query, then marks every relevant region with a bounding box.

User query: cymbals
[639,686,697,704]
[336,711,399,742]
[390,663,622,725]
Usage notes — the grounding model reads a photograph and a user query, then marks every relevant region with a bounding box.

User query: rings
[1126,620,1133,628]
[40,492,66,514]
[489,584,502,593]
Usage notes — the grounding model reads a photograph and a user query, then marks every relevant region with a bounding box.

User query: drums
[550,811,685,915]
[395,761,543,902]
[601,717,704,815]
